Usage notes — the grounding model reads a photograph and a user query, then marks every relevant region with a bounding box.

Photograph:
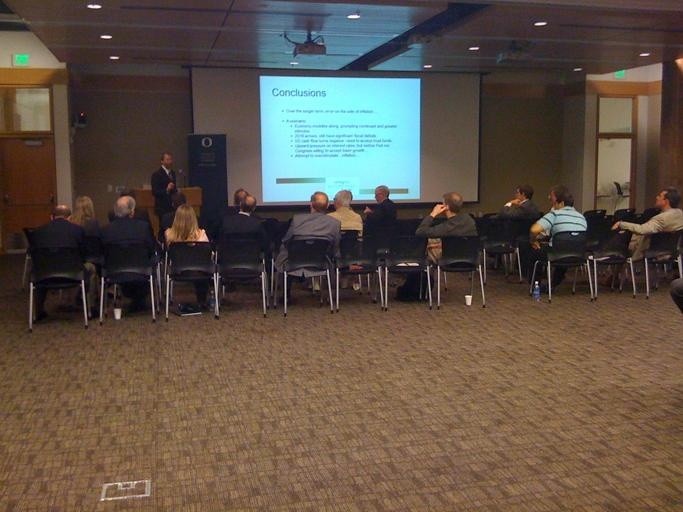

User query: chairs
[614,181,630,210]
[22,209,483,313]
[527,211,682,303]
[484,213,526,284]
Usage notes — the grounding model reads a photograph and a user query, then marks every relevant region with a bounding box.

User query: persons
[273,186,397,303]
[28,187,273,322]
[487,186,683,314]
[397,192,480,303]
[151,152,176,227]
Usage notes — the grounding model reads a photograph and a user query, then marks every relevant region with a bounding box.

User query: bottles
[210,291,215,311]
[533,281,540,301]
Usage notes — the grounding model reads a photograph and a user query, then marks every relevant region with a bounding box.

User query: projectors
[293,43,327,62]
[494,52,534,68]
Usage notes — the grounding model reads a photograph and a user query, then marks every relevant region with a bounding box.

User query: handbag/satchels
[397,272,434,301]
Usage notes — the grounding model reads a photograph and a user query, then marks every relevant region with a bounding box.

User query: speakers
[186,133,228,243]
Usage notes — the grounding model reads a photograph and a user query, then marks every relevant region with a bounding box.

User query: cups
[465,295,472,306]
[113,308,122,320]
